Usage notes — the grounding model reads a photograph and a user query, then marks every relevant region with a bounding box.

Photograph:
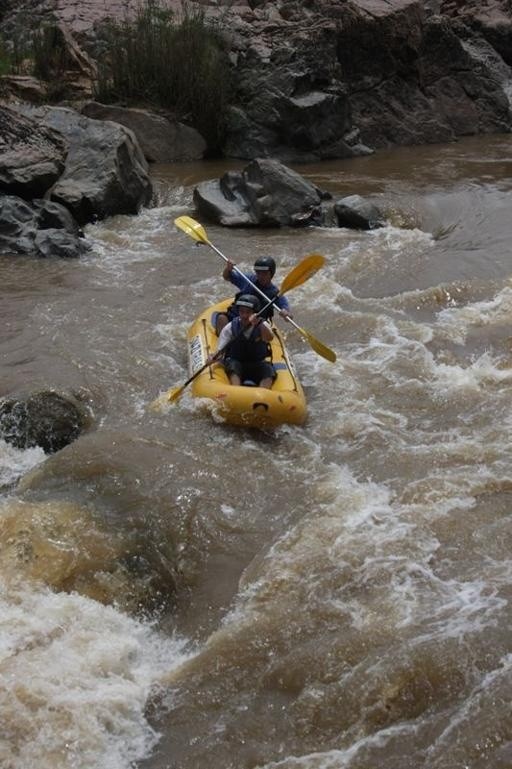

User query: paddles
[173,215,337,364]
[150,252,329,413]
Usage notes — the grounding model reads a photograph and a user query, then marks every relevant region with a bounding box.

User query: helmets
[236,295,261,317]
[254,255,275,278]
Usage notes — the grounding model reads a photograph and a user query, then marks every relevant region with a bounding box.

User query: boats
[187,297,308,430]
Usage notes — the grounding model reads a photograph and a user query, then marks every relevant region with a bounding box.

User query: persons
[209,295,277,390]
[216,256,294,338]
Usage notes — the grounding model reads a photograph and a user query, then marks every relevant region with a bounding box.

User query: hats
[254,265,270,271]
[236,301,253,308]
